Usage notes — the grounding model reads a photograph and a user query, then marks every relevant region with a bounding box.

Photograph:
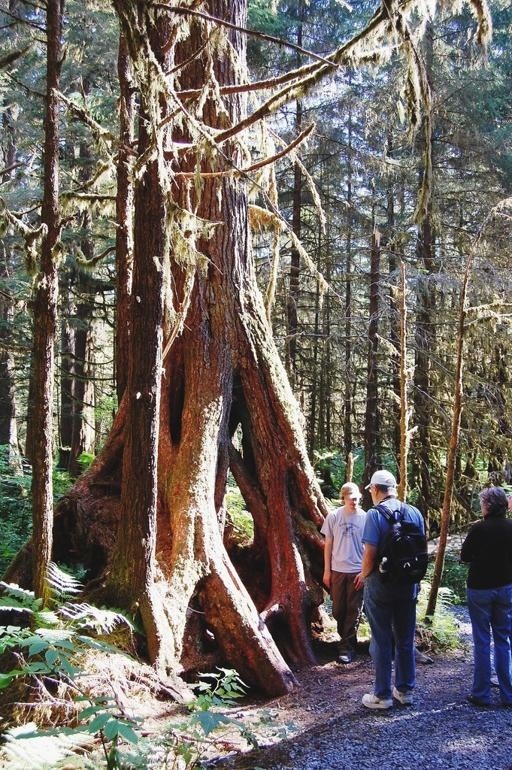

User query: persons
[458,487,512,710]
[320,482,368,663]
[361,468,426,710]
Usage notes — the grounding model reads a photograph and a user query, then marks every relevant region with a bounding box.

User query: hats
[341,482,362,499]
[364,470,399,490]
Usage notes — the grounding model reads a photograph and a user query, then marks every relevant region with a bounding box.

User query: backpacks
[373,502,428,588]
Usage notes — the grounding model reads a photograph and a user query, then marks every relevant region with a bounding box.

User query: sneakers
[392,686,413,705]
[468,695,488,707]
[339,639,356,663]
[362,693,393,709]
